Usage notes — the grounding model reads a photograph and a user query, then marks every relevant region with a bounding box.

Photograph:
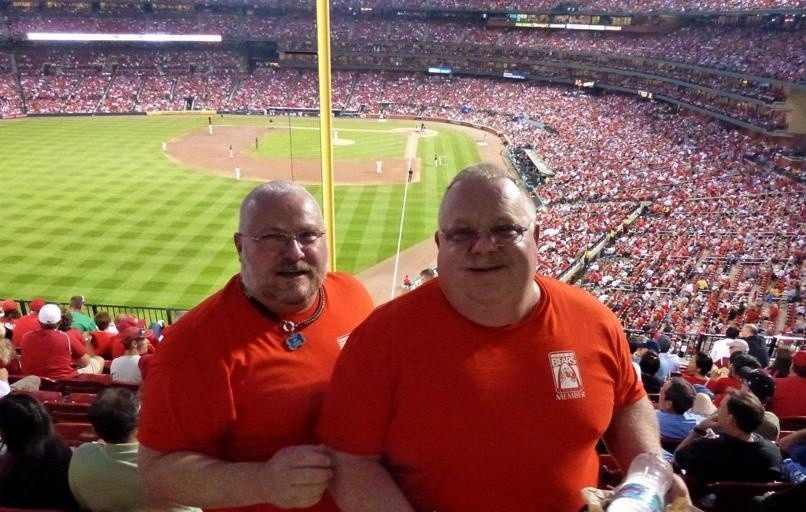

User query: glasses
[437,219,535,249]
[238,228,326,247]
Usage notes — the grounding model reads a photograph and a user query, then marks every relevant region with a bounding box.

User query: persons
[0,295,164,396]
[376,159,383,176]
[163,141,168,151]
[68,385,203,512]
[209,124,212,134]
[1,392,80,511]
[402,132,806,512]
[0,0,805,131]
[137,181,374,512]
[313,162,691,512]
[255,136,258,148]
[229,144,232,158]
[235,167,240,178]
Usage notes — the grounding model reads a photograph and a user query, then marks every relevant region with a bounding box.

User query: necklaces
[240,280,326,350]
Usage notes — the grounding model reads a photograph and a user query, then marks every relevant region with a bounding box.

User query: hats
[37,303,62,326]
[115,314,165,345]
[0,298,46,313]
[639,332,672,354]
[752,411,780,443]
[788,348,806,369]
[739,364,778,404]
[691,392,718,416]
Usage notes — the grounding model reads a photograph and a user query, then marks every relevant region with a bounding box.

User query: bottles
[605,450,675,512]
